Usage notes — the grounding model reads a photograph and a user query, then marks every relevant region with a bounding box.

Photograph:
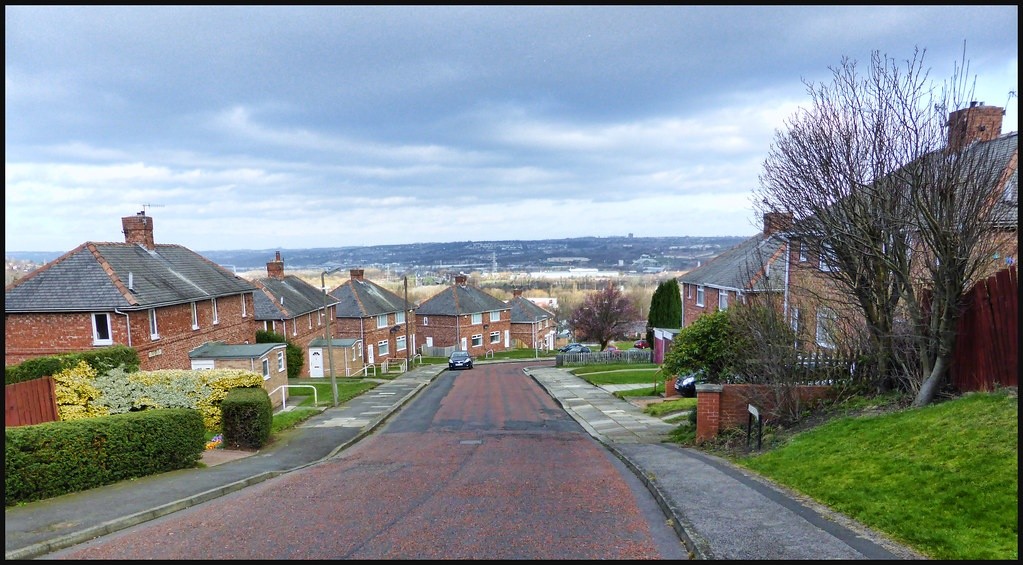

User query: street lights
[321,265,342,407]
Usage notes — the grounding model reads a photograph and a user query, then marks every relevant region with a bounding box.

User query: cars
[674,366,707,397]
[557,343,583,353]
[564,346,592,362]
[634,340,650,349]
[602,345,623,359]
[448,350,474,371]
